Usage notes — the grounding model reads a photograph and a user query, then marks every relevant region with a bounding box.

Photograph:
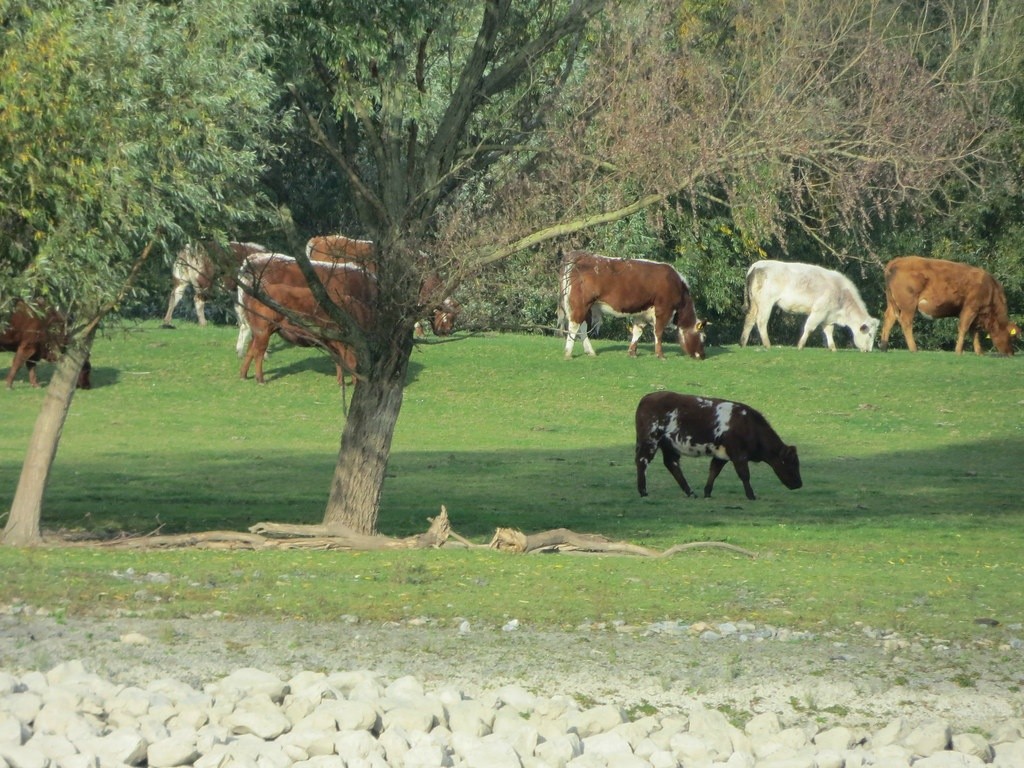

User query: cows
[0,296,91,390]
[880,256,1021,357]
[635,391,802,500]
[739,259,880,353]
[556,250,713,360]
[163,235,459,384]
